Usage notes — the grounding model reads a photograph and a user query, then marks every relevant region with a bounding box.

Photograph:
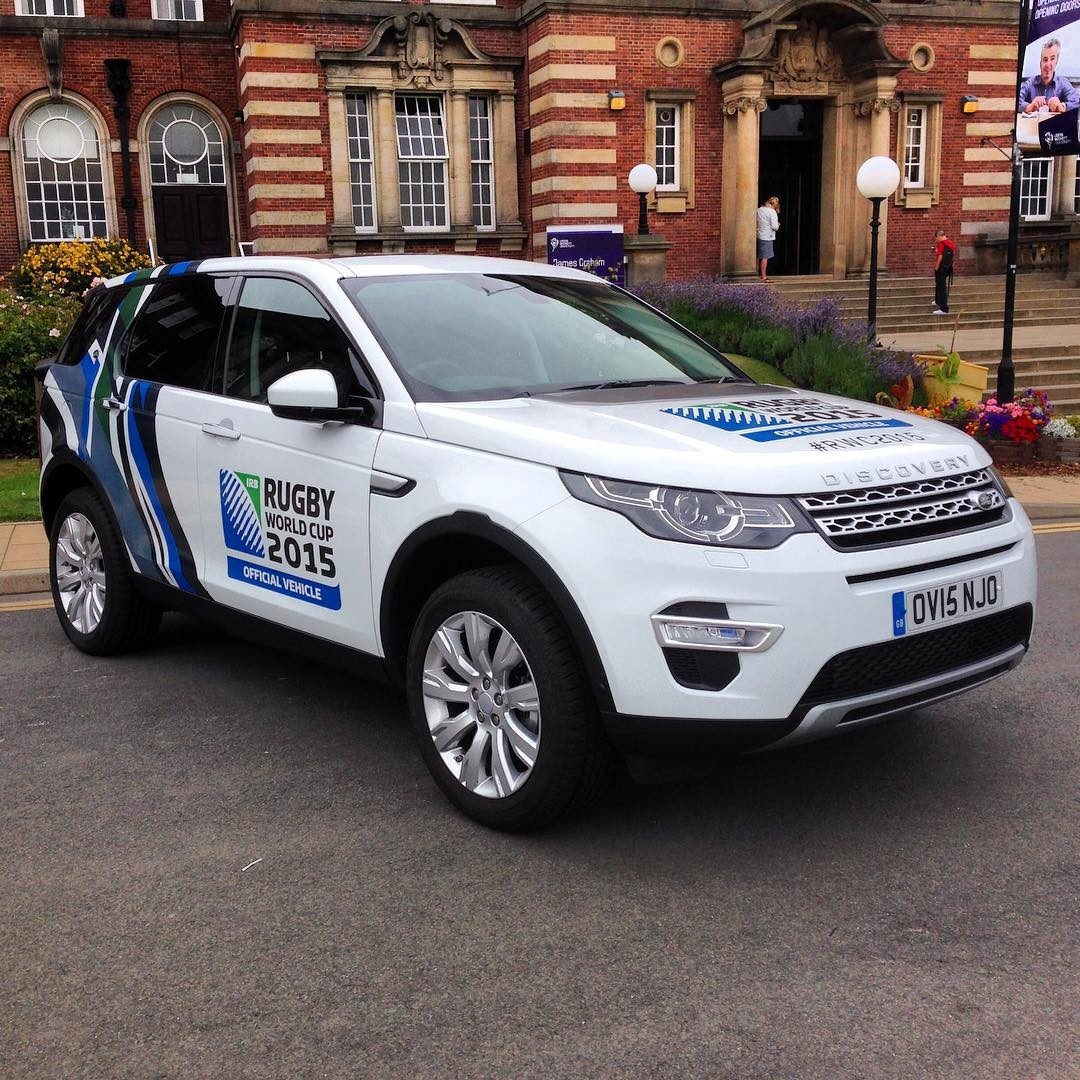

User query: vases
[943,415,1080,469]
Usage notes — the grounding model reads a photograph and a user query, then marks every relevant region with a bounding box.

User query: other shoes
[931,301,938,305]
[933,310,947,314]
[760,277,774,283]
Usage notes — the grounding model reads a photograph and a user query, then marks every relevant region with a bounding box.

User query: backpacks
[940,241,954,277]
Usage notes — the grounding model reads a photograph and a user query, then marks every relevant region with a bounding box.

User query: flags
[1016,1,1080,159]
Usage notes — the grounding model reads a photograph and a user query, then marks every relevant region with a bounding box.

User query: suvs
[32,256,1038,836]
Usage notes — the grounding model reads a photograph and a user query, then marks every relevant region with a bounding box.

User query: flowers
[900,385,1080,442]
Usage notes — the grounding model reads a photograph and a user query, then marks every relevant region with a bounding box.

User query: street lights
[856,156,900,346]
[630,163,657,233]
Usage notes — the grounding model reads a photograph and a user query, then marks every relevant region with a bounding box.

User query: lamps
[608,90,625,110]
[960,96,978,113]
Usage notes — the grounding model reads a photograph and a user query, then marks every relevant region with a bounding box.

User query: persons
[756,196,781,280]
[1018,38,1080,113]
[931,230,956,314]
